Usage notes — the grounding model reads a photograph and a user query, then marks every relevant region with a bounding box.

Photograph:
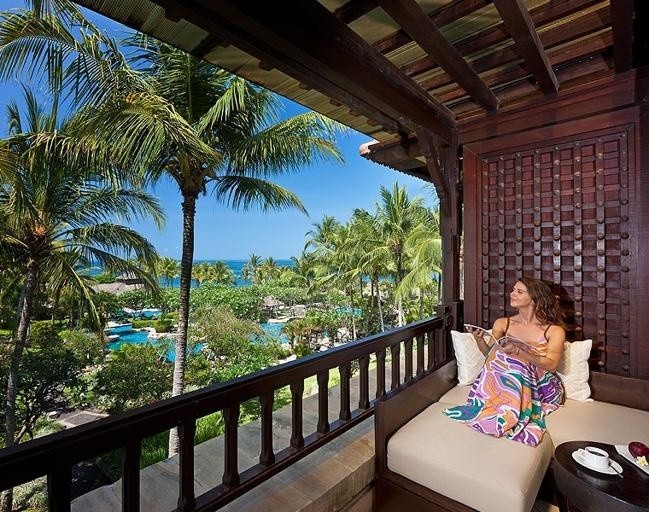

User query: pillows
[449,328,496,387]
[556,337,594,403]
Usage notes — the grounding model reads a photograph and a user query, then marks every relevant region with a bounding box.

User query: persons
[439,276,569,448]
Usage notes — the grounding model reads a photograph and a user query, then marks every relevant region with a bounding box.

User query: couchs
[373,355,649,512]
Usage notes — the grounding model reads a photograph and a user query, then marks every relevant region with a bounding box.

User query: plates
[572,450,623,477]
[615,443,649,476]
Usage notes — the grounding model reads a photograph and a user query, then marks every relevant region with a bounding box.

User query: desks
[551,437,649,512]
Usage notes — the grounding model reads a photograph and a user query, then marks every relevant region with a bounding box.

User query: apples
[629,442,649,463]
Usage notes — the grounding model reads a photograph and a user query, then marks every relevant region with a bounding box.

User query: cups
[577,446,610,468]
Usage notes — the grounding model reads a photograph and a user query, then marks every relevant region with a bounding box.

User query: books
[462,322,537,353]
